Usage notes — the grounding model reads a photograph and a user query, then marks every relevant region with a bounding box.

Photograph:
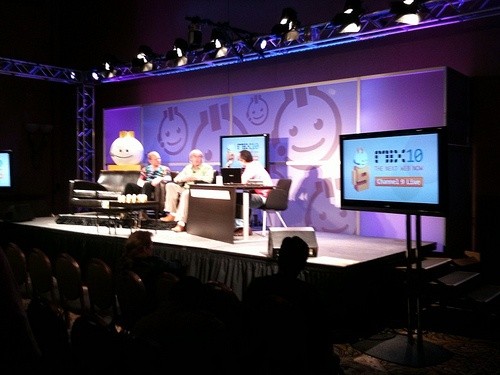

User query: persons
[275,236,310,282]
[125,230,156,280]
[124,150,173,228]
[235,150,274,236]
[160,150,214,232]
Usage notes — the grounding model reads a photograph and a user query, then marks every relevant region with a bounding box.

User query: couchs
[68,170,185,214]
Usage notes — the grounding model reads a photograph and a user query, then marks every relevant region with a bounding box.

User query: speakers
[268,227,318,257]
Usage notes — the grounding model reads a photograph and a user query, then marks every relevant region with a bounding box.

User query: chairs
[257,179,292,236]
[0,243,247,375]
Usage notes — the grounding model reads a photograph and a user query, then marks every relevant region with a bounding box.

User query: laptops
[222,168,241,184]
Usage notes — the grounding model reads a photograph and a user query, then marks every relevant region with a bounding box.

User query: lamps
[330,0,367,34]
[132,47,159,73]
[166,37,191,67]
[390,0,431,24]
[271,9,302,42]
[91,57,122,80]
[204,29,231,59]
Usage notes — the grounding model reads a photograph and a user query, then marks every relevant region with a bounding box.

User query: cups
[116,194,148,203]
[216,175,223,186]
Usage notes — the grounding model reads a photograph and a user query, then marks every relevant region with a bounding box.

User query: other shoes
[171,224,186,232]
[159,214,175,222]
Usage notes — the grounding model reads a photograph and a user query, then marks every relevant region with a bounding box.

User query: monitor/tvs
[219,133,269,169]
[340,126,449,217]
[0,149,13,188]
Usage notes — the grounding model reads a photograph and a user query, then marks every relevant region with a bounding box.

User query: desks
[108,201,160,234]
[187,184,273,244]
